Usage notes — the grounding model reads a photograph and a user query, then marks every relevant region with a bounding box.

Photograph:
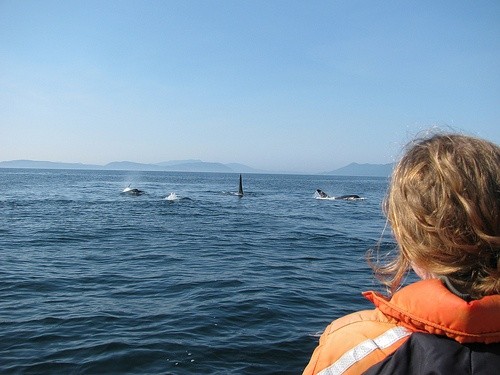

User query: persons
[302,127,500,375]
